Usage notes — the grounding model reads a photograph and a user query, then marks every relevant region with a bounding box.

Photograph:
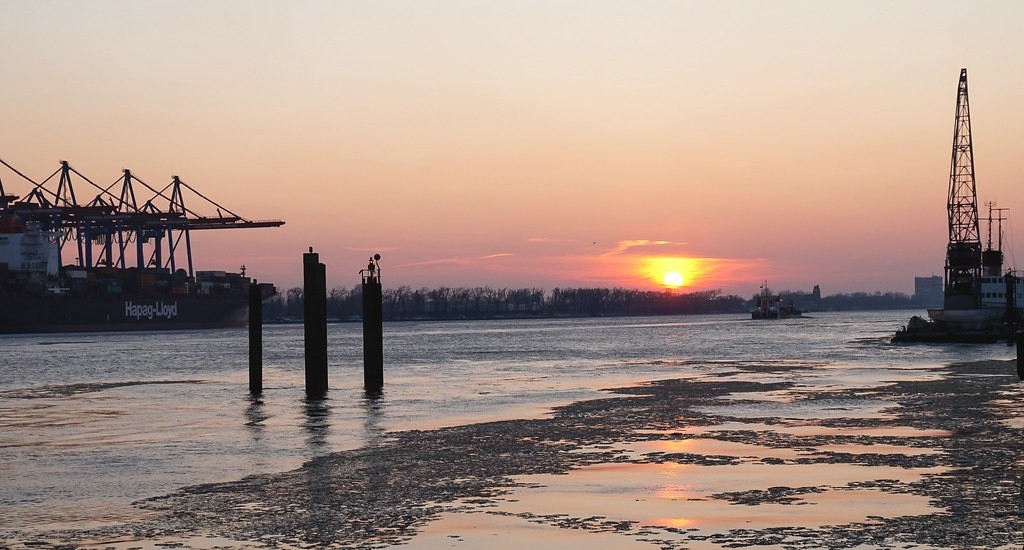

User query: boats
[749,281,810,319]
[925,60,1024,324]
[0,148,286,330]
